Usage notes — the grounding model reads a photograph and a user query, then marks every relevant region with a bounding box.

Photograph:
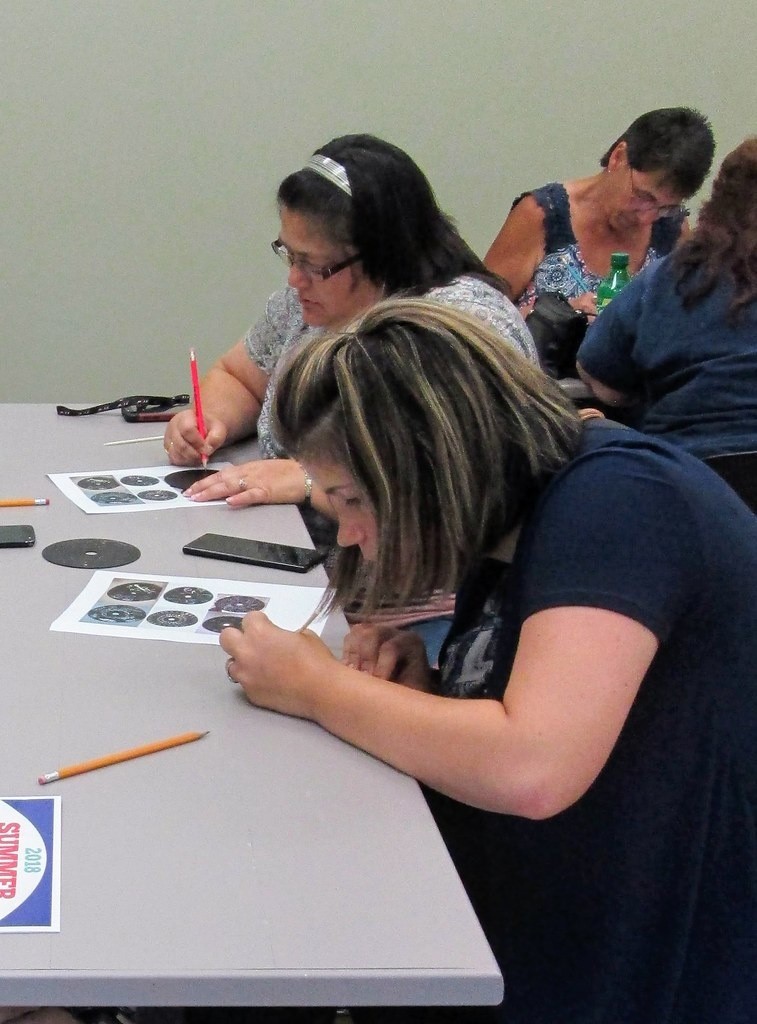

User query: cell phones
[182,532,327,573]
[0,525,36,549]
[121,401,193,423]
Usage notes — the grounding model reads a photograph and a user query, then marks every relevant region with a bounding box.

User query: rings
[239,479,247,491]
[164,441,174,455]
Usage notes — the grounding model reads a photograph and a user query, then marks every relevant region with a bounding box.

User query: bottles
[597,253,633,316]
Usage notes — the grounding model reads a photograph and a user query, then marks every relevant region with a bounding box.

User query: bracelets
[303,462,313,508]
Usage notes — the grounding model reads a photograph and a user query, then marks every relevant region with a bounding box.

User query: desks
[0,401,504,1023]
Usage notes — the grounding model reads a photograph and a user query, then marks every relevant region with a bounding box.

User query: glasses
[629,166,686,219]
[272,239,365,281]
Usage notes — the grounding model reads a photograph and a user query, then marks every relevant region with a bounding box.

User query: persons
[479,104,757,521]
[161,104,542,668]
[215,294,757,1024]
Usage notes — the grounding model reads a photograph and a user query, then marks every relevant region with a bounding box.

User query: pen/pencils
[0,497,50,508]
[104,436,164,446]
[188,346,209,468]
[561,257,588,293]
[38,730,213,785]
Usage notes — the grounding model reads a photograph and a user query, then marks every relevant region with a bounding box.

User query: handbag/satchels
[525,292,589,380]
